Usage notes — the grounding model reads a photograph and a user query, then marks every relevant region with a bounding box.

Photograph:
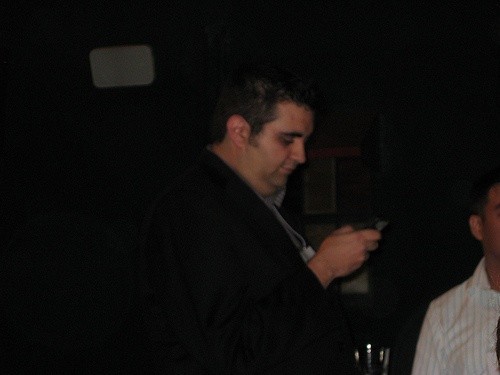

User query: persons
[139,63,382,375]
[409,168,500,375]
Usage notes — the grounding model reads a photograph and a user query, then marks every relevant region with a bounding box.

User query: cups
[353,343,392,375]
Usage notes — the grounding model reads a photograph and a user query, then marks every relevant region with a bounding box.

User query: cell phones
[353,214,390,233]
